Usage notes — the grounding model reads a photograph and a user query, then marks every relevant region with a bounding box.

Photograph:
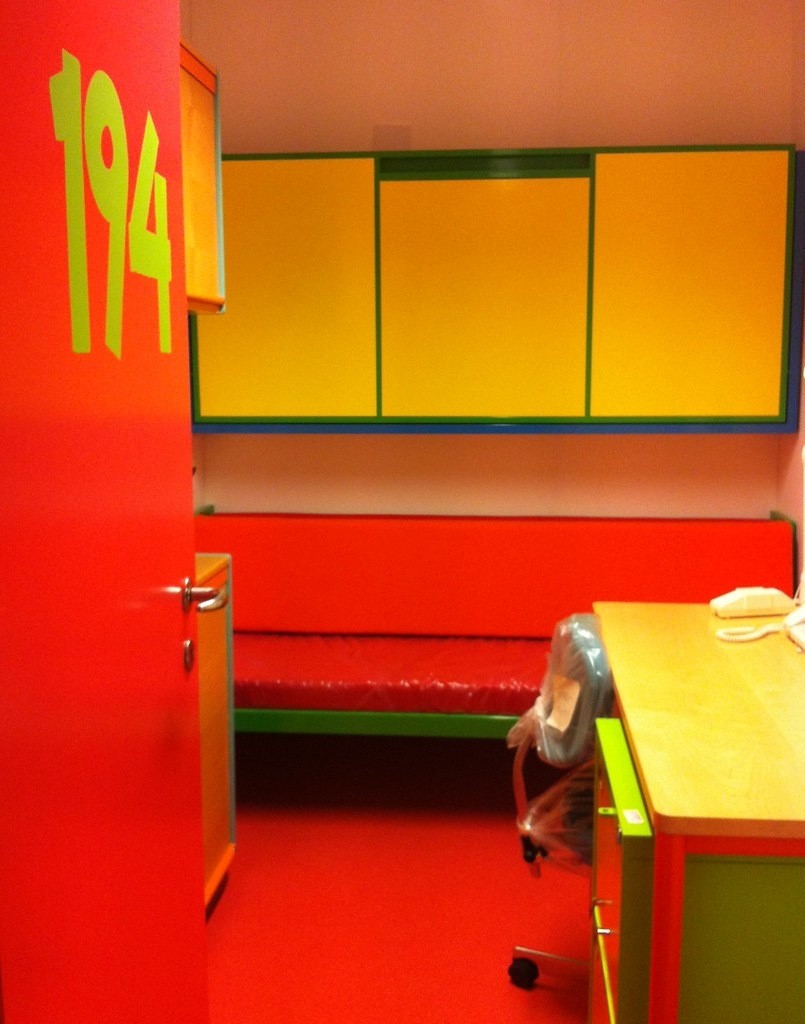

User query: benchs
[196,504,799,740]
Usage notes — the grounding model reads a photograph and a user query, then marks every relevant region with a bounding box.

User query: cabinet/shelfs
[184,144,799,425]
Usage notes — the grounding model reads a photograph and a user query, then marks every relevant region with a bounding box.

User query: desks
[585,599,805,1024]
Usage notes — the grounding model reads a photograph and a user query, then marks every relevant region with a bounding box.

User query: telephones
[782,603,805,653]
